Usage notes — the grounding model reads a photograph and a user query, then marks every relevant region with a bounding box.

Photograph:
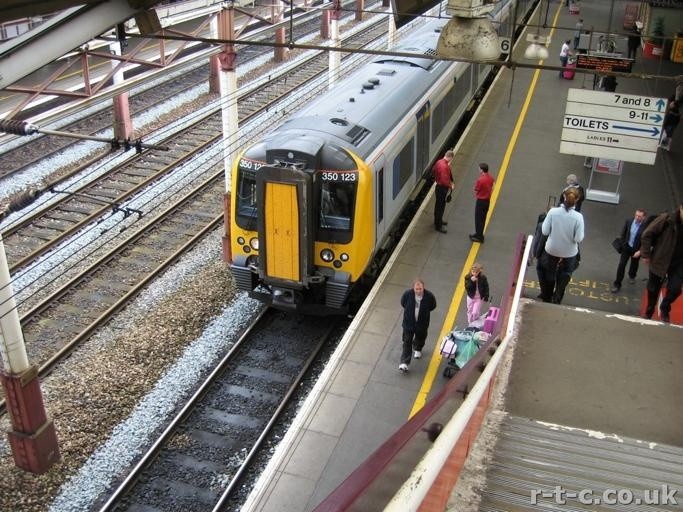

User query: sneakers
[414,351,421,358]
[611,287,618,292]
[661,311,668,322]
[399,364,409,371]
[469,234,484,242]
[628,277,635,283]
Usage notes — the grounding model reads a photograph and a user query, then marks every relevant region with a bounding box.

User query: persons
[465,263,488,323]
[573,20,583,50]
[532,175,584,304]
[431,149,455,233]
[469,162,494,243]
[559,39,574,78]
[628,26,641,63]
[658,81,683,152]
[641,204,683,322]
[610,209,646,293]
[399,278,437,373]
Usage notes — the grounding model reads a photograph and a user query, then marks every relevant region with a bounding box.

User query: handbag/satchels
[638,215,669,246]
[612,238,624,253]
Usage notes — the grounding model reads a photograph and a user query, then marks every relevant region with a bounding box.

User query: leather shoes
[442,221,448,225]
[436,227,447,233]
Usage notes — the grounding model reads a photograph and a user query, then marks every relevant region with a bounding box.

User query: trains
[223,1,539,317]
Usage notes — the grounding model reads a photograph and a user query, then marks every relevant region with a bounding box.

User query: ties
[449,167,453,182]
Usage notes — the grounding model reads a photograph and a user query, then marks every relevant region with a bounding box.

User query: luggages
[536,196,556,235]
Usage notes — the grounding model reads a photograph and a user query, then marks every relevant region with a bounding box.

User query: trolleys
[442,325,480,378]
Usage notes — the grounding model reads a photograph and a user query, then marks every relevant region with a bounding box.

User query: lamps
[524,1,549,61]
